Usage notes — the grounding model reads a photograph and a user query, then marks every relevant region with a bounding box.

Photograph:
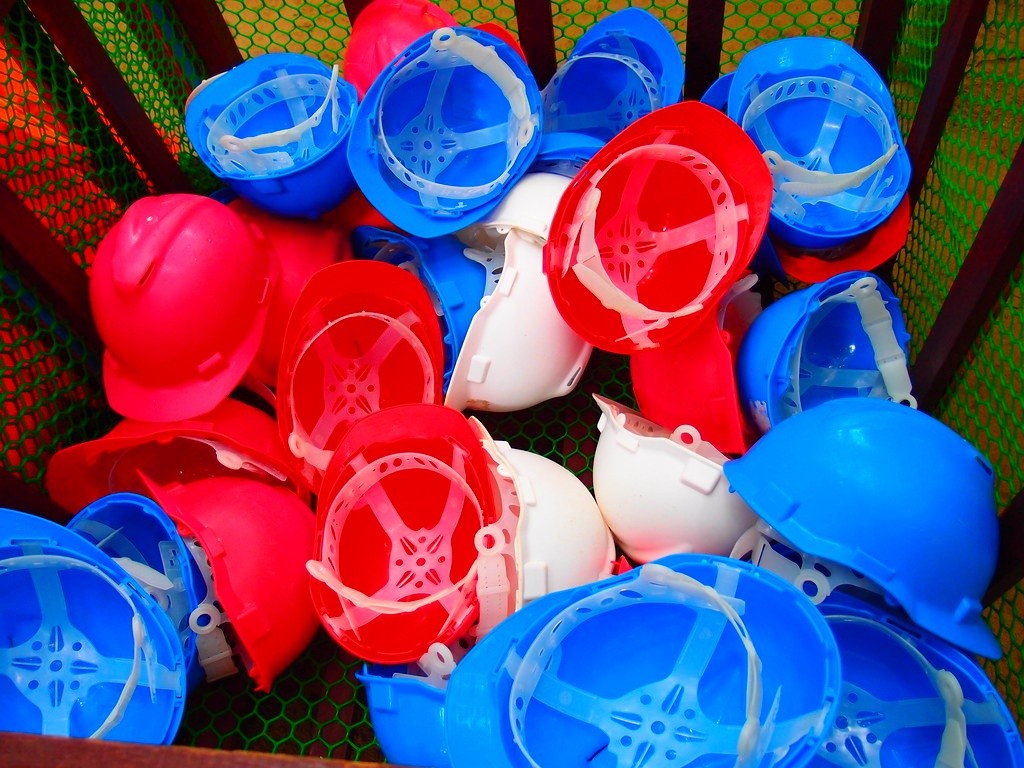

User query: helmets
[1,0,1024,768]
